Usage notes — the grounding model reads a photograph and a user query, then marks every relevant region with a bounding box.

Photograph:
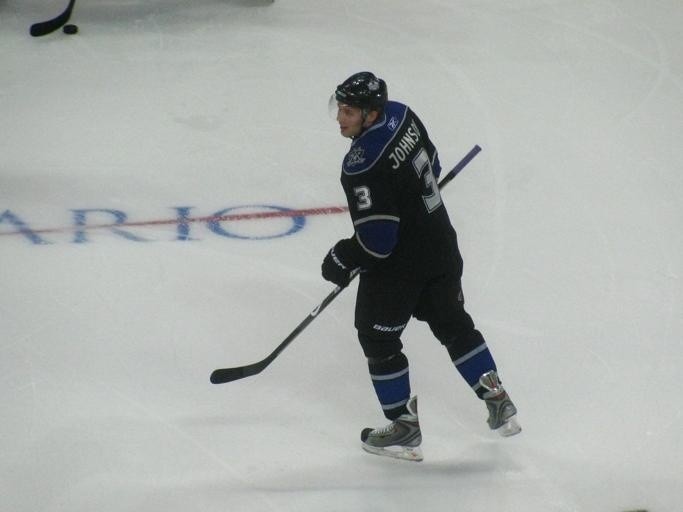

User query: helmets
[329,72,387,120]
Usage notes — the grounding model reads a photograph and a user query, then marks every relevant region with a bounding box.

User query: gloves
[322,239,359,286]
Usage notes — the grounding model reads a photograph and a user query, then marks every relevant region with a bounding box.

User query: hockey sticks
[210,145,480,383]
[30,0,75,37]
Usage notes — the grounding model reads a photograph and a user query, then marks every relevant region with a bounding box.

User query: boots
[361,395,422,447]
[479,370,517,429]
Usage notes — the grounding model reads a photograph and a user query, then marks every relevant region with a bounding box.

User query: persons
[320,70,519,449]
